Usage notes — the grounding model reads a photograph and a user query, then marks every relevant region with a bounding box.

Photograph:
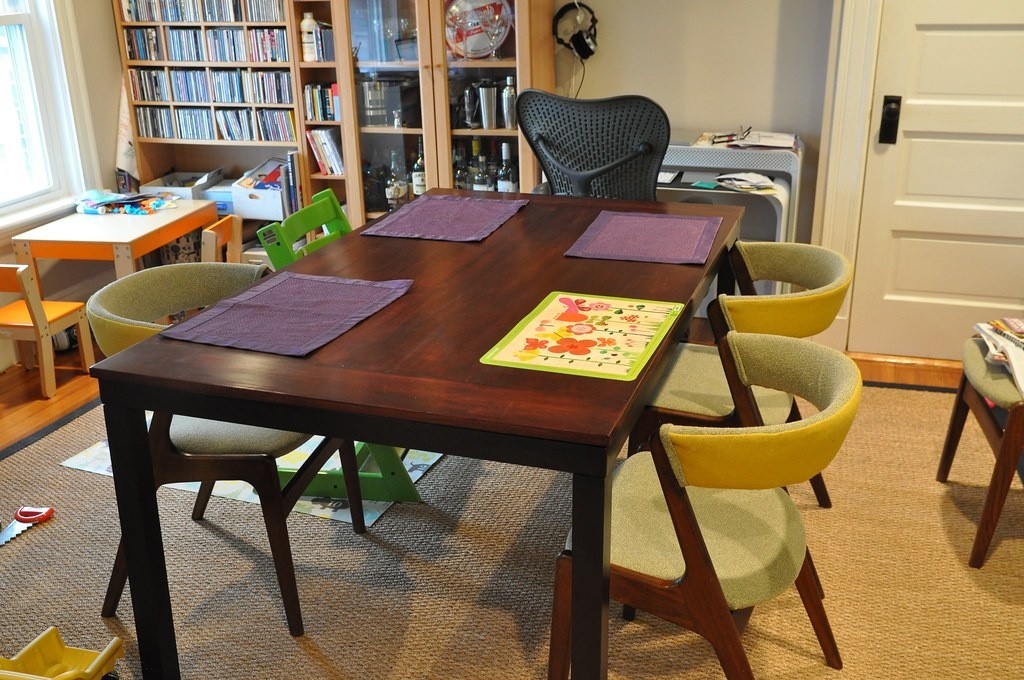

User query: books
[974,317,1024,393]
[121,0,343,221]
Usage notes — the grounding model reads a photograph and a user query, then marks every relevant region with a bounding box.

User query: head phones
[553,2,598,59]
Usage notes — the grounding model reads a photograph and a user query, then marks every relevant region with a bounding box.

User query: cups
[362,81,388,127]
[479,86,498,130]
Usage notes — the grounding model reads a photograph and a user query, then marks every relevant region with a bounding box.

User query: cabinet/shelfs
[290,0,557,246]
[112,0,311,269]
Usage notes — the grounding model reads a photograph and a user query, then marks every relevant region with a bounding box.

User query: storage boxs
[139,157,285,221]
[115,171,139,193]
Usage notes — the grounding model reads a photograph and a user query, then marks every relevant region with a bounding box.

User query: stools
[935,333,1024,569]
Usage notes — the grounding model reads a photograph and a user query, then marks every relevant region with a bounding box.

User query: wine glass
[373,4,515,61]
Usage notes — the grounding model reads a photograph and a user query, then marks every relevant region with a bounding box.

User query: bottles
[299,12,318,62]
[365,134,427,212]
[501,76,519,130]
[452,135,519,193]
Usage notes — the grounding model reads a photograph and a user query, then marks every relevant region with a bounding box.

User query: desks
[90,185,745,680]
[11,198,219,370]
[542,128,806,320]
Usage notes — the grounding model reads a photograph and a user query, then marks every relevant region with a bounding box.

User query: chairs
[255,187,423,504]
[515,89,714,204]
[546,240,863,680]
[0,263,95,399]
[155,211,243,328]
[85,261,367,637]
